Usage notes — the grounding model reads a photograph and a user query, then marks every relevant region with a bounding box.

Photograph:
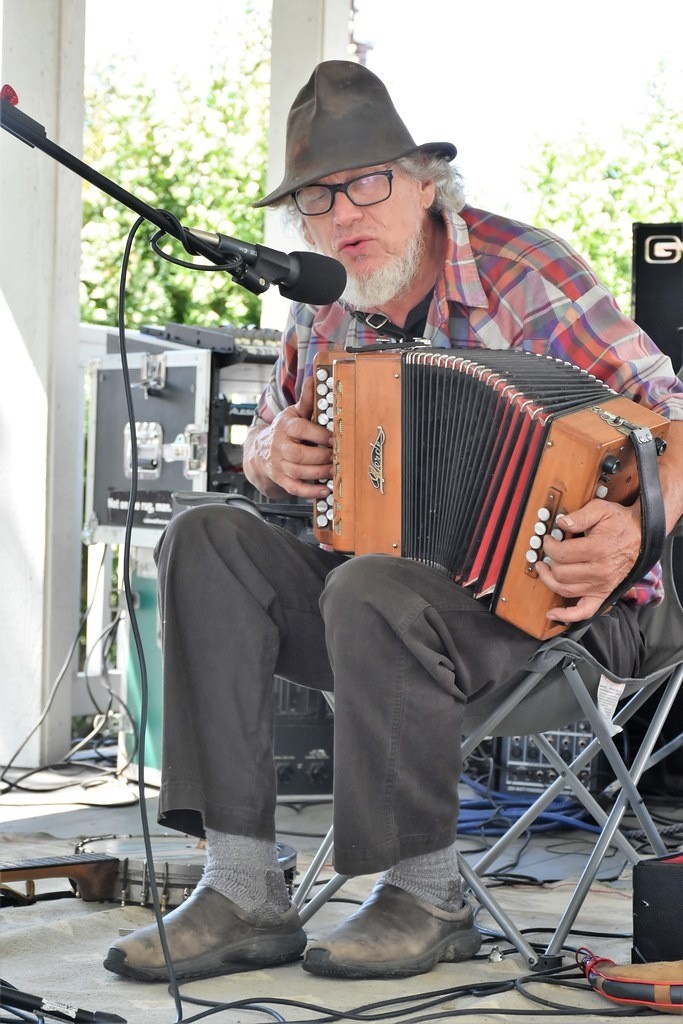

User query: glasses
[292,159,398,215]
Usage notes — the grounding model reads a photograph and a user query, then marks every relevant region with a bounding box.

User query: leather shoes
[301,878,485,978]
[104,884,309,982]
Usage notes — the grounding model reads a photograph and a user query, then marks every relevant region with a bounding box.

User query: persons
[104,60,682,984]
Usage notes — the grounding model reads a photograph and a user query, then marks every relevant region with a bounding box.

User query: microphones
[180,227,347,306]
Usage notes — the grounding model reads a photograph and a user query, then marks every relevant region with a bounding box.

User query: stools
[283,597,683,970]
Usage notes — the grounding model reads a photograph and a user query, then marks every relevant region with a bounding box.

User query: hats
[253,60,457,209]
